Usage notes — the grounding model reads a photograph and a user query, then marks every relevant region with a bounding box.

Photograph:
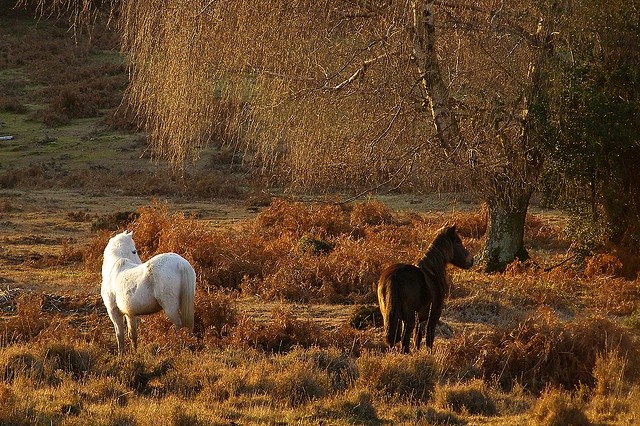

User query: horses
[376,220,475,355]
[99,229,197,357]
